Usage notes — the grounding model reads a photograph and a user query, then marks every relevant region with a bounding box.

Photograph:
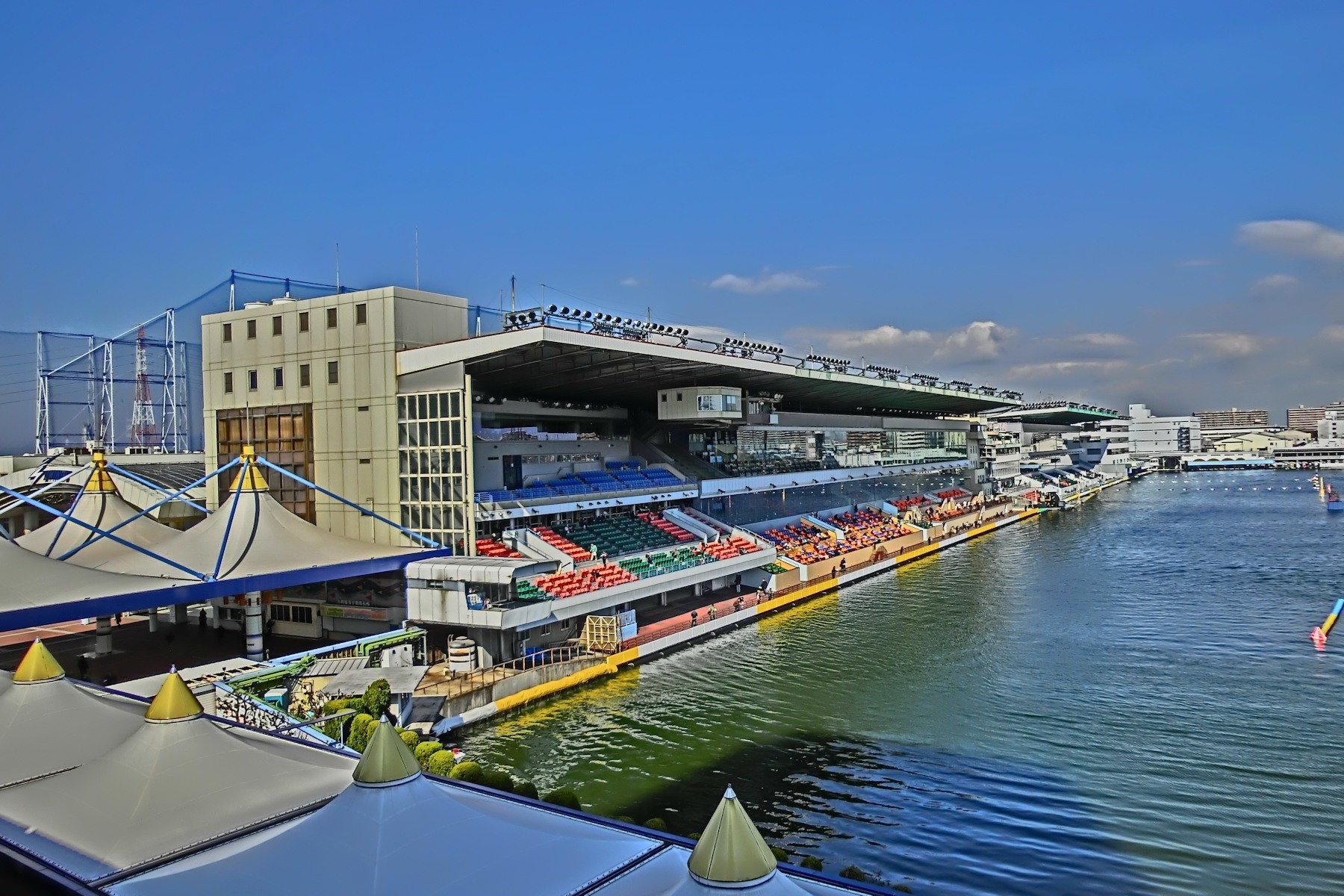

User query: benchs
[477,457,1097,602]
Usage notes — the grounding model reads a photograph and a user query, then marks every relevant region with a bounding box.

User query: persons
[1024,468,1104,494]
[199,608,207,621]
[267,620,274,634]
[116,613,120,625]
[691,489,1027,628]
[77,655,115,687]
[523,507,748,579]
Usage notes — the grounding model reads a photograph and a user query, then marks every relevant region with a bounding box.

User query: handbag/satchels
[839,564,843,568]
[707,609,712,616]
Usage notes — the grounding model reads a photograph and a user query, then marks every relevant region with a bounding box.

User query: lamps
[474,304,1118,414]
